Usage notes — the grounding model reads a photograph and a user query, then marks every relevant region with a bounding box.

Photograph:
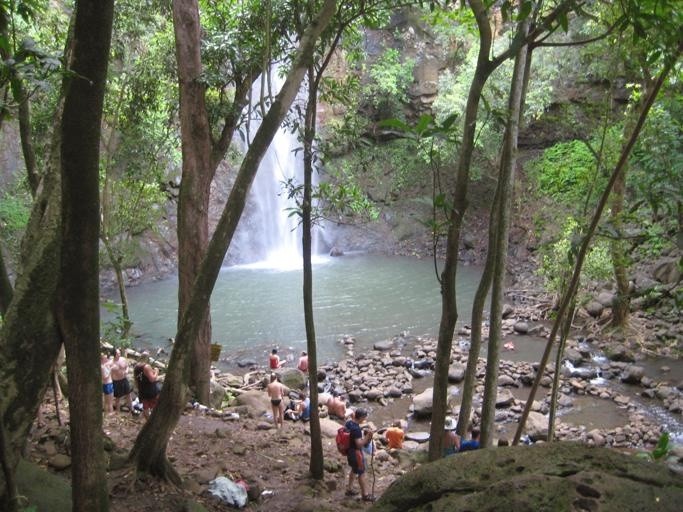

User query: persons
[268,346,287,369]
[326,392,339,416]
[109,348,141,416]
[383,420,404,449]
[288,392,309,422]
[100,354,116,421]
[133,350,159,421]
[266,371,285,431]
[343,407,377,501]
[334,396,355,422]
[456,427,481,453]
[295,351,308,375]
[440,416,459,457]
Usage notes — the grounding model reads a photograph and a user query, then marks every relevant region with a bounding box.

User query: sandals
[129,410,141,415]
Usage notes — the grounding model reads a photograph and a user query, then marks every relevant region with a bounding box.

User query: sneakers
[362,493,377,501]
[345,488,360,496]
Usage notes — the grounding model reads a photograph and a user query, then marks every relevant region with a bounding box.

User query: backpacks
[335,426,352,456]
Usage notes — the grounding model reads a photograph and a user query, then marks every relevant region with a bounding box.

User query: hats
[444,416,458,430]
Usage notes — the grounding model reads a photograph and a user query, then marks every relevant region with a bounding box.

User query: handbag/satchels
[144,380,161,399]
